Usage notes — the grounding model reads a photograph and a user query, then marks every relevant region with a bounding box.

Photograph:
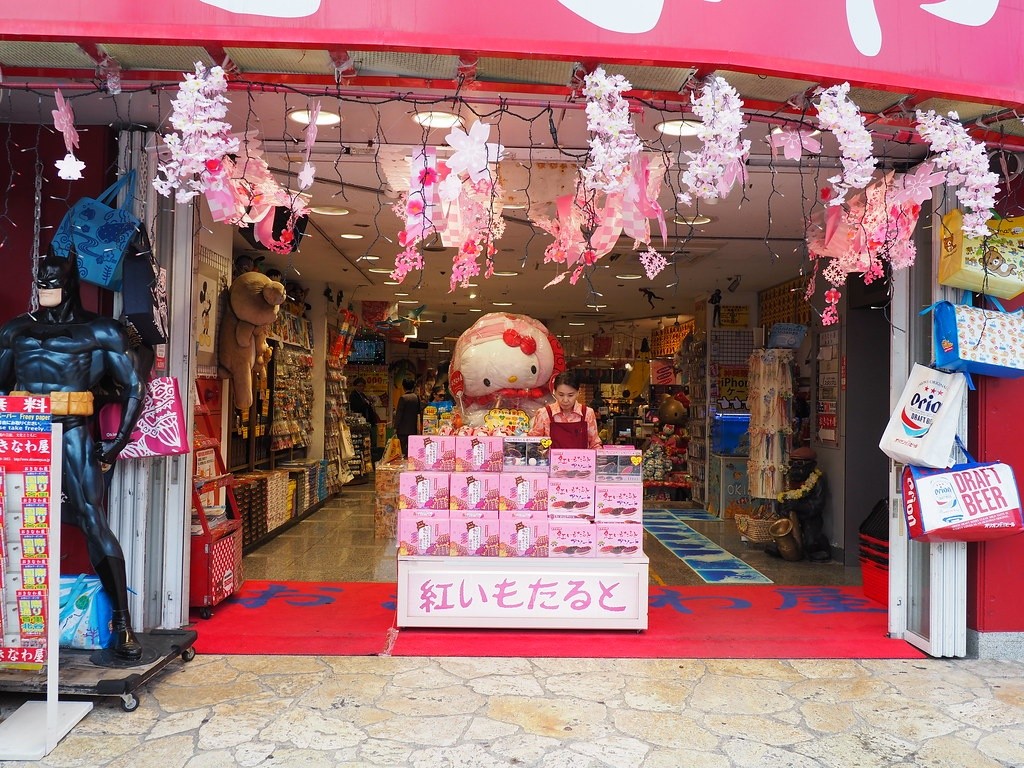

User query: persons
[393,379,421,455]
[350,377,373,424]
[0,256,146,658]
[429,386,445,409]
[528,373,602,450]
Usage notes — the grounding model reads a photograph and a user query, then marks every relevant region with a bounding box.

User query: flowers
[811,81,880,188]
[580,67,643,194]
[915,109,1001,239]
[676,77,751,208]
[150,61,240,203]
[446,164,507,294]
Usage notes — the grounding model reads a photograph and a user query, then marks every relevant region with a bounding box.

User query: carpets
[182,578,928,659]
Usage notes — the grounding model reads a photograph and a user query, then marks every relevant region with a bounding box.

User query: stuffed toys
[449,312,564,427]
[218,272,286,409]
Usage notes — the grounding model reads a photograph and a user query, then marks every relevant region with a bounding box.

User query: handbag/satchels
[938,204,1024,300]
[59,573,117,650]
[100,365,191,460]
[919,288,1024,379]
[50,169,141,292]
[879,362,975,468]
[902,435,1024,543]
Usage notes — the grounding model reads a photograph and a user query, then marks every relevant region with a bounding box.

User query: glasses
[435,392,447,397]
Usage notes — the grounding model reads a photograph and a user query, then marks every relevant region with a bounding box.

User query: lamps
[727,280,739,293]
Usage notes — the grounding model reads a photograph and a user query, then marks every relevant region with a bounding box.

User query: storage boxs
[399,518,450,556]
[596,524,644,558]
[503,436,551,472]
[499,472,548,511]
[374,460,407,498]
[374,497,398,539]
[857,499,889,566]
[499,518,548,558]
[450,471,499,511]
[399,470,449,510]
[449,518,499,557]
[549,448,596,482]
[548,522,596,558]
[713,415,748,457]
[595,485,643,524]
[548,478,595,520]
[407,435,455,472]
[858,559,889,607]
[232,465,318,546]
[455,436,503,472]
[595,449,643,483]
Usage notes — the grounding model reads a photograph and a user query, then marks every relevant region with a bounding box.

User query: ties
[358,393,371,406]
